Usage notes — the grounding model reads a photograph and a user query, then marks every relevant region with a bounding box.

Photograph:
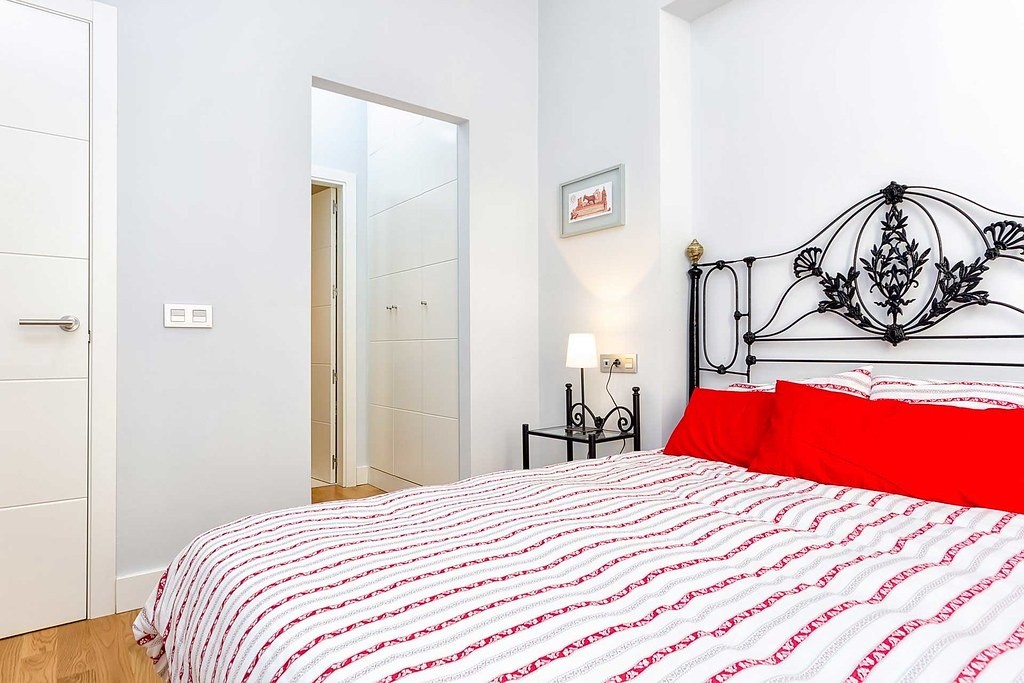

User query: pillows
[746,380,1024,514]
[727,365,873,400]
[869,375,1024,412]
[663,387,776,468]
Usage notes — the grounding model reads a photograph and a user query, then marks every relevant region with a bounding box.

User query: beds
[132,180,1024,683]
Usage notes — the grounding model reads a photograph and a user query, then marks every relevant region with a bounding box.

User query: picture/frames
[559,163,625,239]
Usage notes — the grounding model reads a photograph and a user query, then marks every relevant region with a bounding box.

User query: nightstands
[522,383,641,470]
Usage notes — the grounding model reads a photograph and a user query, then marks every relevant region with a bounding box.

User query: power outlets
[600,354,637,373]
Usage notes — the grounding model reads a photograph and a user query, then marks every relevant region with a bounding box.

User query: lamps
[565,333,604,436]
